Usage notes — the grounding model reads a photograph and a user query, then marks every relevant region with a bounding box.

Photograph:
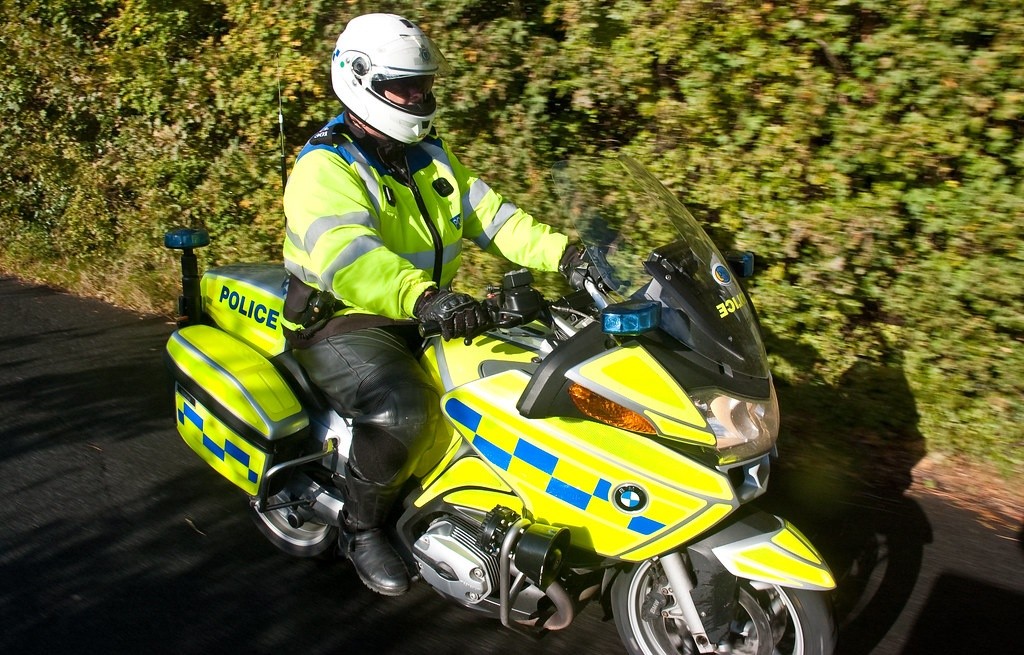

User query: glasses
[376,75,435,100]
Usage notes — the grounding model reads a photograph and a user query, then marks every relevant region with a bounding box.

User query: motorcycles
[161,61,841,655]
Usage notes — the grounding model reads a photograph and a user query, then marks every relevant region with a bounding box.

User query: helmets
[330,12,446,142]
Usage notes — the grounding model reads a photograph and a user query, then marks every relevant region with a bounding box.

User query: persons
[282,13,617,597]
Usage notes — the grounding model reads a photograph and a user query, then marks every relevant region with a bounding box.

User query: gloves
[559,241,622,298]
[413,287,492,342]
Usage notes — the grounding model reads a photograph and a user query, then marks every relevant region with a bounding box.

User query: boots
[336,465,416,597]
